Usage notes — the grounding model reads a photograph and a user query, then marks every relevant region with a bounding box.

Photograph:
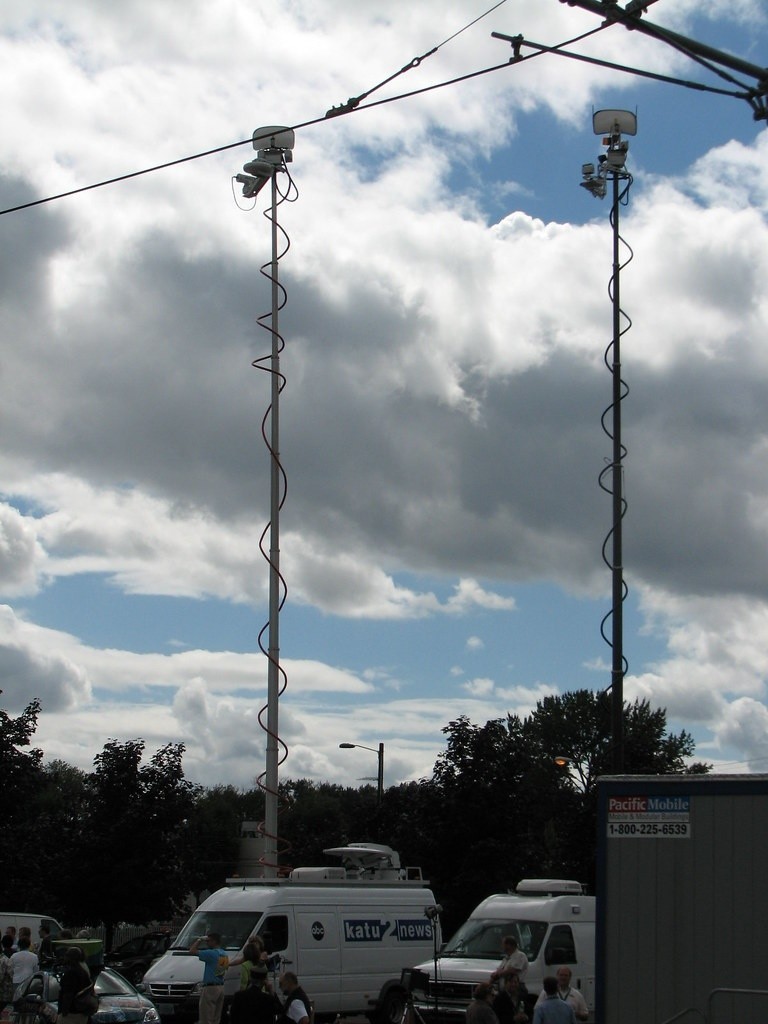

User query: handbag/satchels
[72,994,99,1017]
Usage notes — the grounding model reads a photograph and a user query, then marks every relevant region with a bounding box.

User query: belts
[201,982,224,987]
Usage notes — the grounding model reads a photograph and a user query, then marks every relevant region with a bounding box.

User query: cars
[13,967,165,1024]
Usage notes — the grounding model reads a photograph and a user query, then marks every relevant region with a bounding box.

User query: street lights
[339,743,382,844]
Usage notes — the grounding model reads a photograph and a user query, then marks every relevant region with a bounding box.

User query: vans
[0,912,63,953]
[401,878,596,1024]
[142,841,442,1024]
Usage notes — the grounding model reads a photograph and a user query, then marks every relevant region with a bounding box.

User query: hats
[471,983,494,1003]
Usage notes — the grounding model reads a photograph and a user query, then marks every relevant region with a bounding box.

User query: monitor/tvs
[399,968,430,991]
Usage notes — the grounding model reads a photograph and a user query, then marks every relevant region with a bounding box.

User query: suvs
[104,929,179,987]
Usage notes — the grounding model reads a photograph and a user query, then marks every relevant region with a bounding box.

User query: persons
[466,936,589,1024]
[189,933,314,1024]
[0,924,92,1024]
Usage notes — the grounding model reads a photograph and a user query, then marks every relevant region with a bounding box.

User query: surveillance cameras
[244,161,275,179]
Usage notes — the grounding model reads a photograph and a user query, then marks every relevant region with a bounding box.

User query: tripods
[399,990,428,1024]
